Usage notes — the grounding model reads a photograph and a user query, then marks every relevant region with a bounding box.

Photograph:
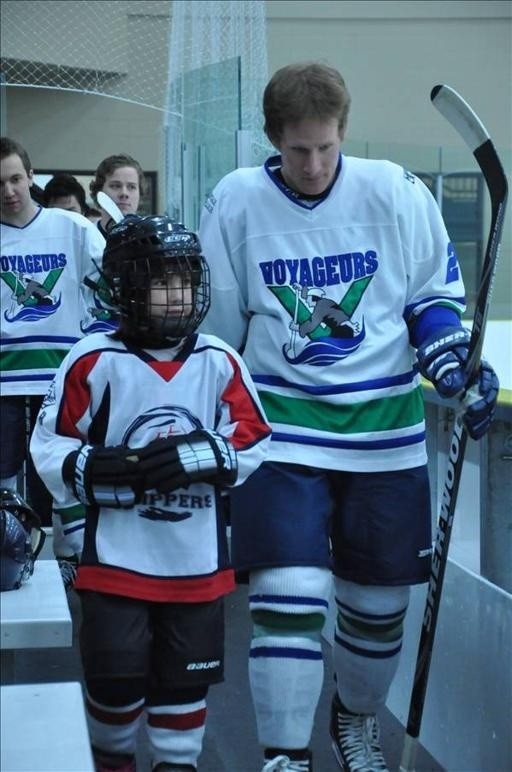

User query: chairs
[415,171,484,292]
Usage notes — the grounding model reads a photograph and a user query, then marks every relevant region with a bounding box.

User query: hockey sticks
[398,85,508,772]
[97,191,125,225]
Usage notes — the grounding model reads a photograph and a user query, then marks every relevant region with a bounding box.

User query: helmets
[101,214,212,339]
[0,488,45,592]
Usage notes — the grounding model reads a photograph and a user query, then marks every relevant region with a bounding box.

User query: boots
[330,674,388,772]
[261,750,310,771]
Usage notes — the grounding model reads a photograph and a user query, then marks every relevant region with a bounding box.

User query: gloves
[139,430,238,495]
[415,328,497,439]
[65,442,146,511]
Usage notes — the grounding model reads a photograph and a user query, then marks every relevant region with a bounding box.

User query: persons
[26,213,277,772]
[0,134,120,536]
[89,152,148,237]
[194,61,502,772]
[43,172,86,218]
[84,207,102,224]
